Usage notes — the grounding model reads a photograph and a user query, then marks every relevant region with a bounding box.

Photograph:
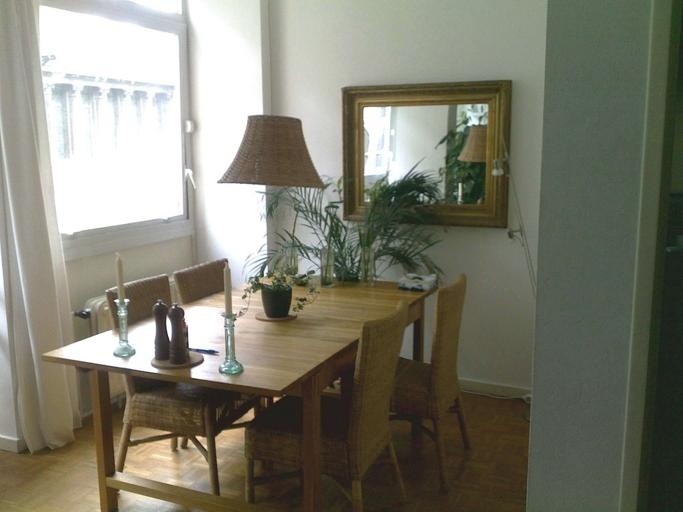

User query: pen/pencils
[190,348,220,354]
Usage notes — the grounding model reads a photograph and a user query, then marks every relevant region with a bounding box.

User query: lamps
[457,124,488,166]
[216,2,324,188]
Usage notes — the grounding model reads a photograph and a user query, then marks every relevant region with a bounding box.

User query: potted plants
[243,259,319,318]
[232,159,446,326]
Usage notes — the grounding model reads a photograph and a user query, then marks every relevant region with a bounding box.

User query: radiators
[74,273,179,413]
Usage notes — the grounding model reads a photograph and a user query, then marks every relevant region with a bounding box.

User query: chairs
[105,274,266,497]
[338,272,470,494]
[174,260,275,407]
[244,298,410,510]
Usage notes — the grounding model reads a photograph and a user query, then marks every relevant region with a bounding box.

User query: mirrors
[353,93,499,218]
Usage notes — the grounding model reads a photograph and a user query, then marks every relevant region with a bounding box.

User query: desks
[39,272,440,511]
